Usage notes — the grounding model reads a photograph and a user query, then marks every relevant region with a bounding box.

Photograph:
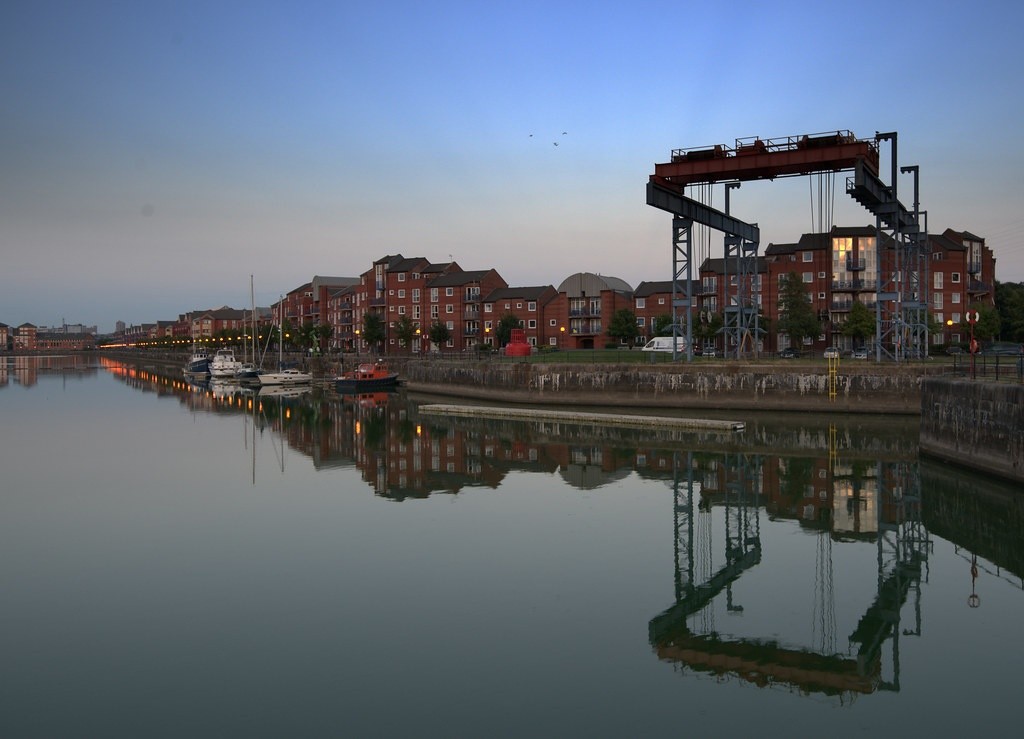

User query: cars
[692,343,704,356]
[824,347,854,360]
[851,346,872,361]
[780,346,800,359]
[945,346,961,357]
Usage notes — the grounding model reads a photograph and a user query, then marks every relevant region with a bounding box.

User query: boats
[332,358,400,390]
[207,349,243,376]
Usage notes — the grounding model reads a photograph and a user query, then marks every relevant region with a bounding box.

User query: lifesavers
[966,310,979,324]
[970,339,978,353]
[330,368,335,374]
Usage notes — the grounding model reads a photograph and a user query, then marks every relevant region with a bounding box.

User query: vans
[702,346,719,359]
[641,337,687,353]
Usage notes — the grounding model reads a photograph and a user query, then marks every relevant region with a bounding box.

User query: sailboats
[258,385,313,474]
[184,319,210,372]
[237,274,263,377]
[256,294,314,385]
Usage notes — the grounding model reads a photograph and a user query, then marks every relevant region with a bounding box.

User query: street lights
[947,320,953,345]
[485,328,489,343]
[561,327,565,349]
[355,329,359,357]
[285,333,289,355]
[416,329,420,359]
